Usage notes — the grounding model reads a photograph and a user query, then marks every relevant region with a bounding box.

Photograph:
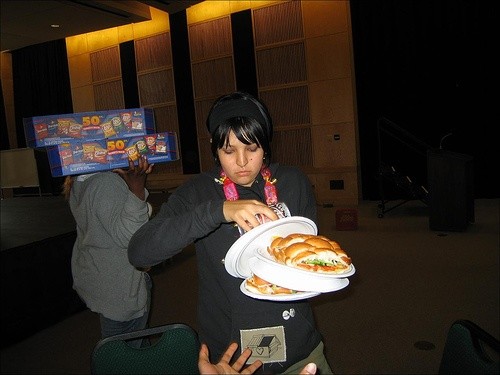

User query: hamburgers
[246,274,297,295]
[266,233,353,275]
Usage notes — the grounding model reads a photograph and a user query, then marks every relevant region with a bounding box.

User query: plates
[239,275,322,301]
[256,245,356,279]
[248,257,350,293]
[224,216,318,279]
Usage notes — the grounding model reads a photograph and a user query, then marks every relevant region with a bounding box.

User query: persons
[63,154,152,341]
[198,343,316,375]
[128,92,334,375]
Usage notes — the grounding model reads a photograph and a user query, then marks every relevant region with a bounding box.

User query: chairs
[437,320,500,375]
[377,163,431,218]
[90,322,200,375]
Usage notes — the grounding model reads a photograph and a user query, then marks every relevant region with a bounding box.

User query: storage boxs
[430,157,475,233]
[336,209,358,231]
